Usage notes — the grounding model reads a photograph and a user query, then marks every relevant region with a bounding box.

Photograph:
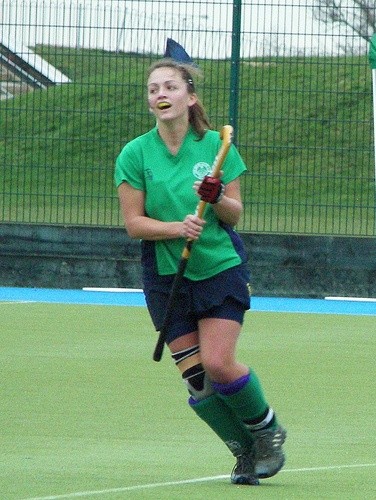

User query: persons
[112,57,287,485]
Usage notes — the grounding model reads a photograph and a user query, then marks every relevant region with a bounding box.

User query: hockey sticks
[153,124,234,364]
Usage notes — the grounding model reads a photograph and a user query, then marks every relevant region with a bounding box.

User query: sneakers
[250,425,286,479]
[230,447,260,486]
